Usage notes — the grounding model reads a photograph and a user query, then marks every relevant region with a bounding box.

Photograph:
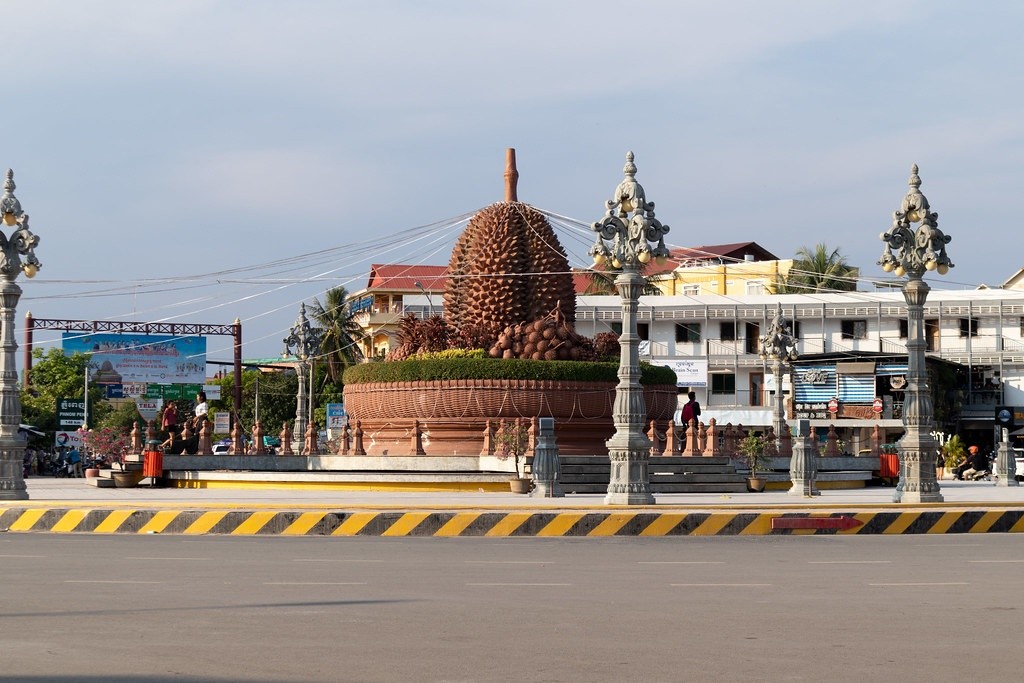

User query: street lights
[0,169,43,500]
[283,301,320,455]
[759,302,799,434]
[878,165,955,504]
[586,151,675,506]
[414,281,438,317]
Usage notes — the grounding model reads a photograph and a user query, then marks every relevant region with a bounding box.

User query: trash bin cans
[880,443,899,476]
[143,440,163,476]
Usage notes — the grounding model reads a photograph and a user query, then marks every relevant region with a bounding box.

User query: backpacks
[682,401,695,422]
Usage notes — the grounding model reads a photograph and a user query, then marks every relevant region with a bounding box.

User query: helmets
[968,446,978,453]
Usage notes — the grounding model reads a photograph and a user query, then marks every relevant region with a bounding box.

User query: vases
[112,473,139,487]
[85,469,99,479]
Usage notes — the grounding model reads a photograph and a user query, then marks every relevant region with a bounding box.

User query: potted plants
[941,434,968,479]
[734,429,775,493]
[485,422,532,494]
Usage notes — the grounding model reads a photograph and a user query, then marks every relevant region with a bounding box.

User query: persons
[161,400,178,448]
[957,446,986,482]
[936,448,946,481]
[680,391,701,431]
[269,446,276,456]
[22,442,87,480]
[190,392,209,446]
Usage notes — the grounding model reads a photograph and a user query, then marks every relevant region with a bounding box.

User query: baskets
[952,469,957,474]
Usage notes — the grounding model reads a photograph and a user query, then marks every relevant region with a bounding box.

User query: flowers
[78,426,133,473]
